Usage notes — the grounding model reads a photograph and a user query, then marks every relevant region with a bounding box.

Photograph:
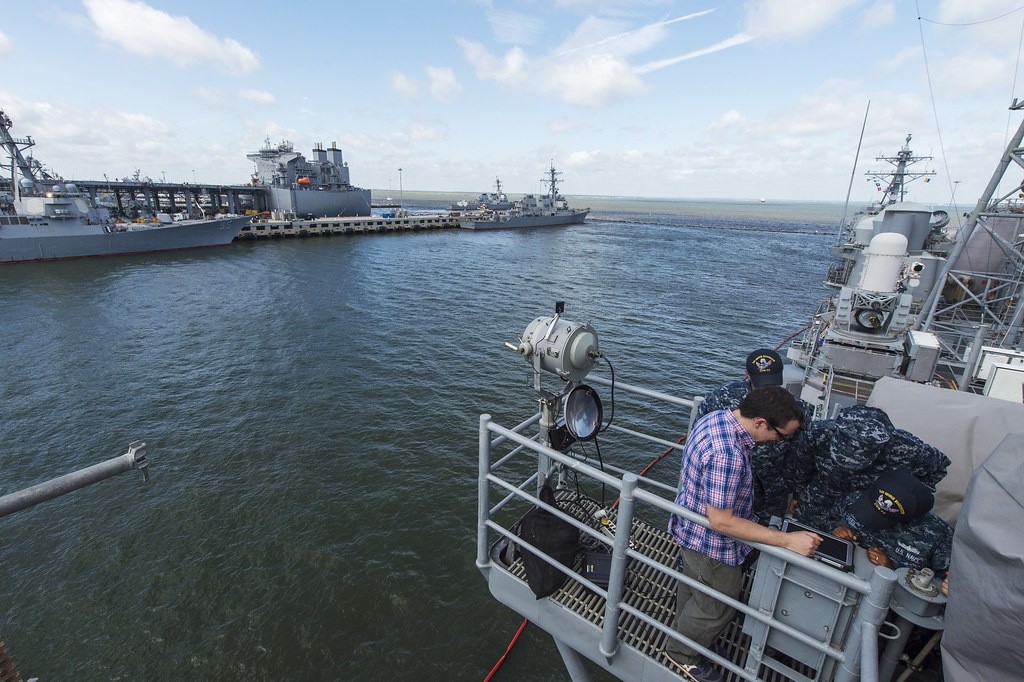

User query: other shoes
[705,643,731,664]
[665,650,722,682]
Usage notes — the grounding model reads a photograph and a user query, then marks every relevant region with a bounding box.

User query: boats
[446,175,519,210]
[0,108,256,265]
[457,152,592,230]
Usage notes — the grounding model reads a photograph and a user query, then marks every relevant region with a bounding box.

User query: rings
[876,556,878,561]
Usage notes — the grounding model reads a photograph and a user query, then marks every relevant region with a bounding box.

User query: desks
[742,514,882,674]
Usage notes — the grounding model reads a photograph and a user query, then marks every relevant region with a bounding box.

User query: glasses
[757,416,793,445]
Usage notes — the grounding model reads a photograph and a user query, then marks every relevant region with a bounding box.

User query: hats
[852,470,935,531]
[830,404,895,470]
[746,349,783,388]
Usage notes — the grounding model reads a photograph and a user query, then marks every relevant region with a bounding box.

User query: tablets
[780,518,854,567]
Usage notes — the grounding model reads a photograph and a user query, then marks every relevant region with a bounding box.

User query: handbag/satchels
[519,485,580,600]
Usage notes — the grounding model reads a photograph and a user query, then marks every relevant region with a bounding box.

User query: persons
[665,386,824,682]
[690,347,957,596]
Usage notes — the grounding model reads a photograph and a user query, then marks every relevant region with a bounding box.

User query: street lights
[399,168,404,216]
